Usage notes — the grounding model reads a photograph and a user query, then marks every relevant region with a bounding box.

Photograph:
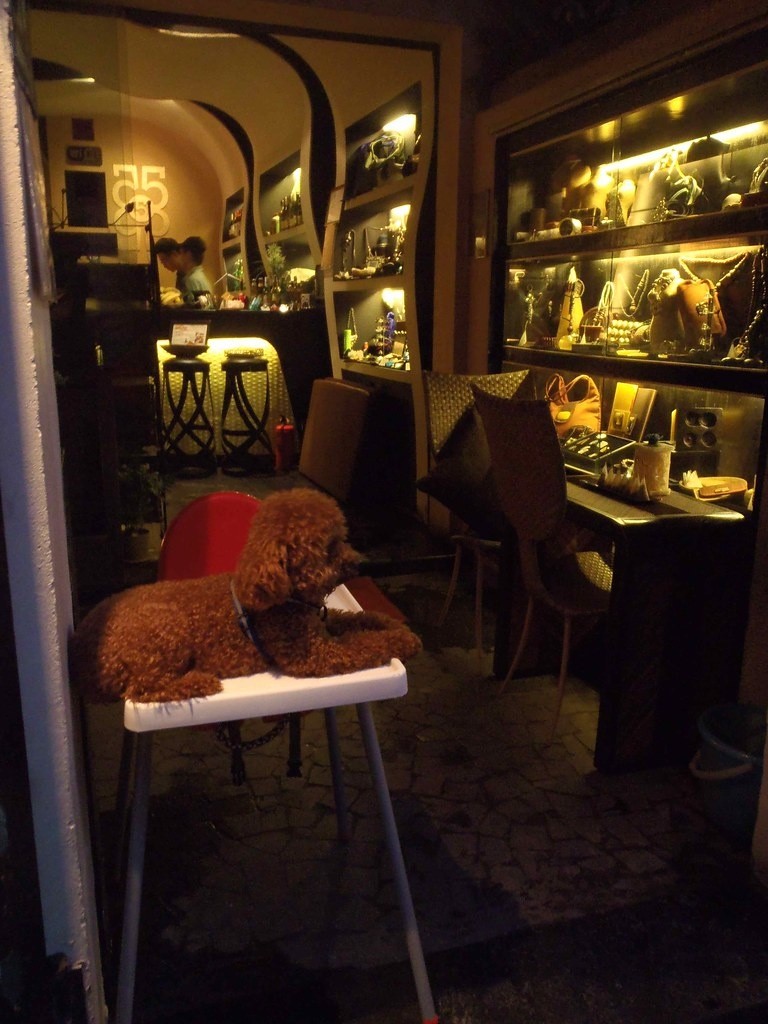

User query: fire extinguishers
[275,416,295,470]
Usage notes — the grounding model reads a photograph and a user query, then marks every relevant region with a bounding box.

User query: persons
[155,235,213,299]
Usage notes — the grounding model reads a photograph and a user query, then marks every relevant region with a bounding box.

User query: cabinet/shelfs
[491,14,768,758]
[217,78,444,506]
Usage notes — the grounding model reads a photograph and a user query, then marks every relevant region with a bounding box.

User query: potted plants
[106,461,175,560]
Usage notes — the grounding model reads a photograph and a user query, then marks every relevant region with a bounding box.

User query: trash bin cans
[686,700,767,833]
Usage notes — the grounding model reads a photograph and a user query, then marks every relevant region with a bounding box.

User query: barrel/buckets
[690,703,765,840]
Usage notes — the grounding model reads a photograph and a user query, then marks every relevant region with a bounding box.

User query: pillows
[414,403,508,542]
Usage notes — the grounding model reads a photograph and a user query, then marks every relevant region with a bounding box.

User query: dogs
[68,487,423,703]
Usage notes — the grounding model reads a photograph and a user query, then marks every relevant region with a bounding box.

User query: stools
[107,493,439,1024]
[217,357,275,470]
[159,356,214,470]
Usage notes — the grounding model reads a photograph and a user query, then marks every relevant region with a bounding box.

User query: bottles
[633,433,672,495]
[223,209,241,242]
[270,193,302,235]
[249,274,319,311]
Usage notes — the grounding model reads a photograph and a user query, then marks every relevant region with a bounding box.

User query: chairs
[468,376,611,750]
[420,366,533,648]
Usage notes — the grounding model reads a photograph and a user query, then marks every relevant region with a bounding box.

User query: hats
[176,236,206,251]
